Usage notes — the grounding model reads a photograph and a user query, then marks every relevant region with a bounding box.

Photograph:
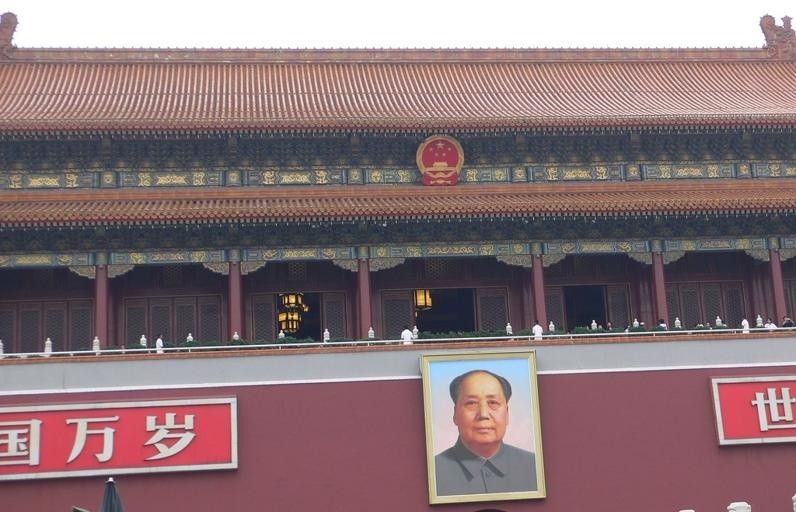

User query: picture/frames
[418,348,547,505]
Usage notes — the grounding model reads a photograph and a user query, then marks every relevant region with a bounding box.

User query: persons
[532,320,543,340]
[156,334,164,353]
[765,318,777,328]
[655,319,667,331]
[741,315,749,333]
[401,325,414,344]
[782,317,794,327]
[435,370,537,496]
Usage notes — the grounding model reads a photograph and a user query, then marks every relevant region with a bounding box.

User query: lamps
[411,288,433,312]
[277,290,310,335]
